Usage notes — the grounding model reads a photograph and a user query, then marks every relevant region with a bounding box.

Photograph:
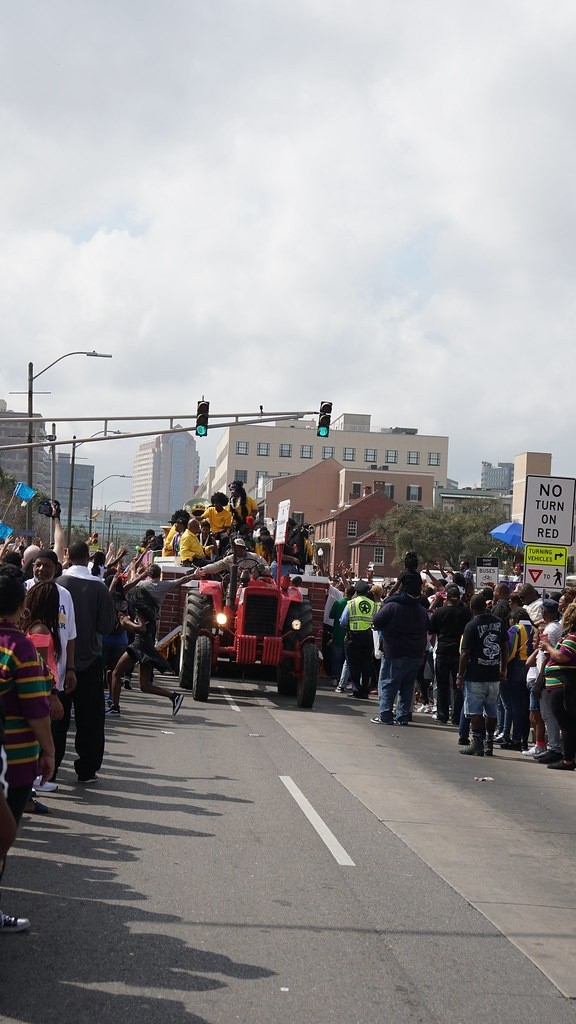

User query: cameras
[40,502,52,515]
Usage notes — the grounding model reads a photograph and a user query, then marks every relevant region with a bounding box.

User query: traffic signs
[522,544,568,590]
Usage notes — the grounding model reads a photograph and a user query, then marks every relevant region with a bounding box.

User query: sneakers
[32,776,58,792]
[0,911,30,933]
[105,705,121,717]
[171,692,184,716]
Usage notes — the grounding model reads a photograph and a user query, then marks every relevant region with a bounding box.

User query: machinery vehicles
[156,534,333,708]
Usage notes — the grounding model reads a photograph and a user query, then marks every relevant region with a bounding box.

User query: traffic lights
[316,400,333,438]
[195,400,211,437]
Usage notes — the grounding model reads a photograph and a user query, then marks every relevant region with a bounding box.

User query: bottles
[270,561,277,582]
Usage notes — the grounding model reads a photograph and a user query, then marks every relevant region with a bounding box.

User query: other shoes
[410,700,458,726]
[124,679,132,690]
[393,718,408,726]
[492,734,563,764]
[547,760,576,770]
[78,774,99,783]
[336,687,346,693]
[370,717,394,724]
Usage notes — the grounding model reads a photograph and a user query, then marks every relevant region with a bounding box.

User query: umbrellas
[489,522,525,563]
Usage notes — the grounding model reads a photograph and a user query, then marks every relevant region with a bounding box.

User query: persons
[318,553,576,772]
[0,498,206,937]
[255,516,315,585]
[224,481,259,541]
[195,491,233,558]
[200,537,272,581]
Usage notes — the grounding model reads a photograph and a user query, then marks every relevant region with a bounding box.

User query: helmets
[191,505,205,514]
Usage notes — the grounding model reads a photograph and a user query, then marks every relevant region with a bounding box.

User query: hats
[539,599,560,613]
[232,538,245,547]
[33,550,58,567]
[168,517,188,526]
[354,580,369,594]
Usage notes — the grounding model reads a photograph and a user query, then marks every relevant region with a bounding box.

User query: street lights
[65,430,131,546]
[101,500,135,549]
[106,512,132,553]
[24,349,113,547]
[86,474,133,537]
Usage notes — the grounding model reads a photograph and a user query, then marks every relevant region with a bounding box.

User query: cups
[539,634,549,642]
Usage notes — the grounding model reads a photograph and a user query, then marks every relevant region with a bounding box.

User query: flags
[0,522,14,539]
[15,482,37,501]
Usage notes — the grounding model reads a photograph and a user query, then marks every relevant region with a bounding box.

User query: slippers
[24,798,51,815]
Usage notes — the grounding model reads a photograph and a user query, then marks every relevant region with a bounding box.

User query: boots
[460,737,484,756]
[483,739,493,756]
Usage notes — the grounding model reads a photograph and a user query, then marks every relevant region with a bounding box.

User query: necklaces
[230,496,241,508]
